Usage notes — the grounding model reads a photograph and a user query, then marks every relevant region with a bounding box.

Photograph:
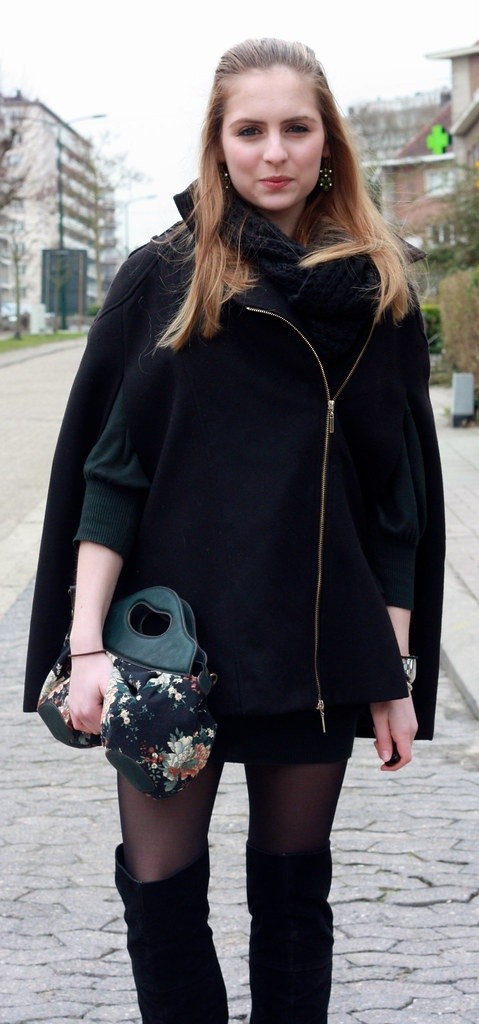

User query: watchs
[400,655,416,691]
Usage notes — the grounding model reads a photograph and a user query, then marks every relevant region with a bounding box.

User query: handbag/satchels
[36,587,219,801]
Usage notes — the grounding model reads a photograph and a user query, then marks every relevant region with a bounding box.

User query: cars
[0,301,29,331]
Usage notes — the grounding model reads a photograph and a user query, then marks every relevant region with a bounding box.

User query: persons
[22,36,446,1024]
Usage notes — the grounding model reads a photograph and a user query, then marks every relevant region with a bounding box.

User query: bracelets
[69,648,107,658]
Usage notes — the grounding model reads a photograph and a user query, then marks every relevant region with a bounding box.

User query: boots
[114,844,229,1024]
[246,841,333,1024]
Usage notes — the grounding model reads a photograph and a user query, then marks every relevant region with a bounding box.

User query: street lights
[113,193,159,261]
[11,112,109,329]
